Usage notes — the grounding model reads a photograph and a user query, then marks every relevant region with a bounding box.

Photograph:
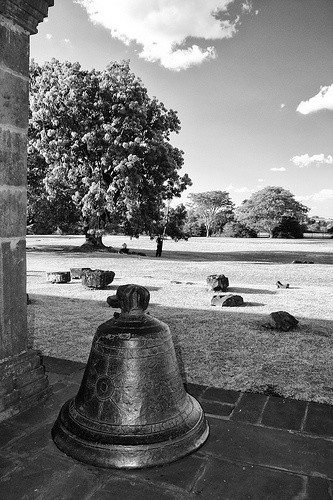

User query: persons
[155,234,164,257]
